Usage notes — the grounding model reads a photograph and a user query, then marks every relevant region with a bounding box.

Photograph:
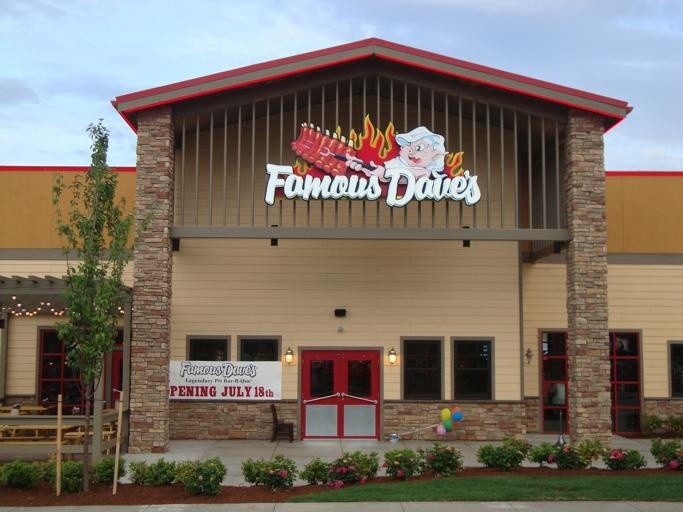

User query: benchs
[0,395,121,454]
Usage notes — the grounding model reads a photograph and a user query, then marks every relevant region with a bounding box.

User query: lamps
[283,347,294,365]
[522,346,534,364]
[387,346,396,363]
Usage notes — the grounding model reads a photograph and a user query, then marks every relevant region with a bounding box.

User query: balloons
[435,408,462,435]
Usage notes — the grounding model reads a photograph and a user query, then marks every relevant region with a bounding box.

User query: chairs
[269,404,293,444]
